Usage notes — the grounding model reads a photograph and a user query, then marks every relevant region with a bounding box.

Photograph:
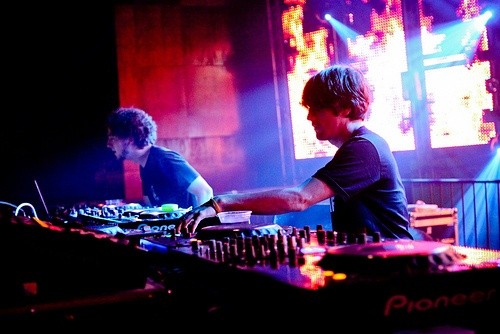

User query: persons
[107,107,214,211]
[175,66,425,243]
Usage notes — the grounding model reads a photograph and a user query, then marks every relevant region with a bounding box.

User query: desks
[408,208,459,246]
